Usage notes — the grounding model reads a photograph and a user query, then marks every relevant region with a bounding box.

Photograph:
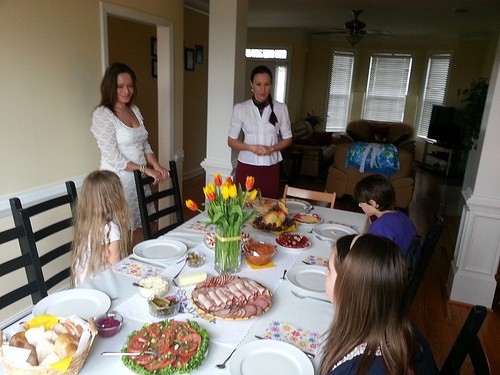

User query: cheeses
[179,271,207,285]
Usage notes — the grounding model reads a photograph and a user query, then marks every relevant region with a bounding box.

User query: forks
[314,235,330,241]
[290,290,333,305]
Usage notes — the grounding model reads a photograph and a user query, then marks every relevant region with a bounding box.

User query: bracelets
[140,164,145,173]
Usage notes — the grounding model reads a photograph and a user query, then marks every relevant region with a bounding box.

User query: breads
[9,321,83,366]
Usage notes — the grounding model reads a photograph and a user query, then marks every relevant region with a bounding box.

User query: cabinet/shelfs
[421,140,459,176]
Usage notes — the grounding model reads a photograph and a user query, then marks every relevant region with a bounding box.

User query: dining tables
[0,196,371,375]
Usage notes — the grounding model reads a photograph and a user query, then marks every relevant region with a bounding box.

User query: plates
[275,233,312,250]
[312,224,359,242]
[229,339,315,375]
[190,276,273,320]
[32,287,111,322]
[286,264,328,294]
[293,213,323,225]
[120,321,208,375]
[282,198,312,214]
[133,239,187,261]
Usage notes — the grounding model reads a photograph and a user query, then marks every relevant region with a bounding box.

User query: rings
[157,179,160,182]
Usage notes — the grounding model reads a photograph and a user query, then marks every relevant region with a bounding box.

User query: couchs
[327,119,415,208]
[291,120,343,176]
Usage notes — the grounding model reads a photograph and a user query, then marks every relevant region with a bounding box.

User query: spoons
[280,270,287,280]
[216,348,237,369]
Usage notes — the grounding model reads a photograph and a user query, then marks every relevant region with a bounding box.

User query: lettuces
[122,318,209,375]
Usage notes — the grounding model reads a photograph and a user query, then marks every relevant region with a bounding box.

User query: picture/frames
[151,35,204,79]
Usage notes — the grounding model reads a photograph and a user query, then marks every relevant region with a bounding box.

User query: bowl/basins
[242,240,276,266]
[185,251,206,268]
[147,294,180,318]
[93,311,123,338]
[138,275,171,299]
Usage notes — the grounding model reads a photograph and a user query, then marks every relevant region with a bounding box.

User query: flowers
[186,175,258,238]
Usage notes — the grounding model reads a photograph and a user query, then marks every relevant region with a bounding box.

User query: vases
[214,228,242,276]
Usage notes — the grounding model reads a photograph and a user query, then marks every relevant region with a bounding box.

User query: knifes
[254,335,315,358]
[302,260,312,265]
[128,257,166,270]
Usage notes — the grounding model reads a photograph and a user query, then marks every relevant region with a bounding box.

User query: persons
[69,170,134,289]
[228,65,294,199]
[90,64,169,256]
[354,174,422,272]
[319,234,442,375]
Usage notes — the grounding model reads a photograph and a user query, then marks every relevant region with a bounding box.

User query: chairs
[0,198,37,329]
[134,160,182,242]
[439,306,491,375]
[10,181,79,304]
[283,185,338,209]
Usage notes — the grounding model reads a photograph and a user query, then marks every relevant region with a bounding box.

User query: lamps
[345,31,363,46]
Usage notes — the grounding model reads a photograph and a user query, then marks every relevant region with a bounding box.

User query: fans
[310,9,394,49]
